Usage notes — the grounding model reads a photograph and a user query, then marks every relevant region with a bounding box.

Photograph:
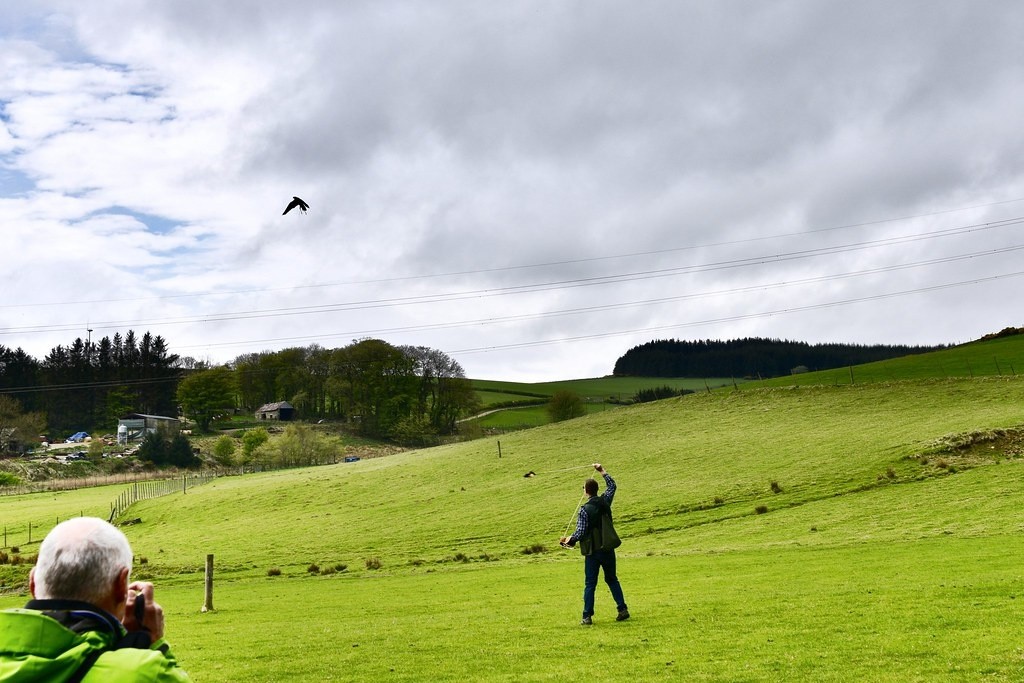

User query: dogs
[523,471,535,478]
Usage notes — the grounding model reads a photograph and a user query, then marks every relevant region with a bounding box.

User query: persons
[0,516,192,683]
[560,463,630,625]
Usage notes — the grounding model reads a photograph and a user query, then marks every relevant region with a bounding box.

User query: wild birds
[282,196,311,217]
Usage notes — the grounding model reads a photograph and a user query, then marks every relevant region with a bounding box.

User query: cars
[66,451,90,460]
[68,431,90,443]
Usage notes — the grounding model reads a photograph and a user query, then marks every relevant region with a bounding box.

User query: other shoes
[616,610,630,620]
[581,617,592,625]
